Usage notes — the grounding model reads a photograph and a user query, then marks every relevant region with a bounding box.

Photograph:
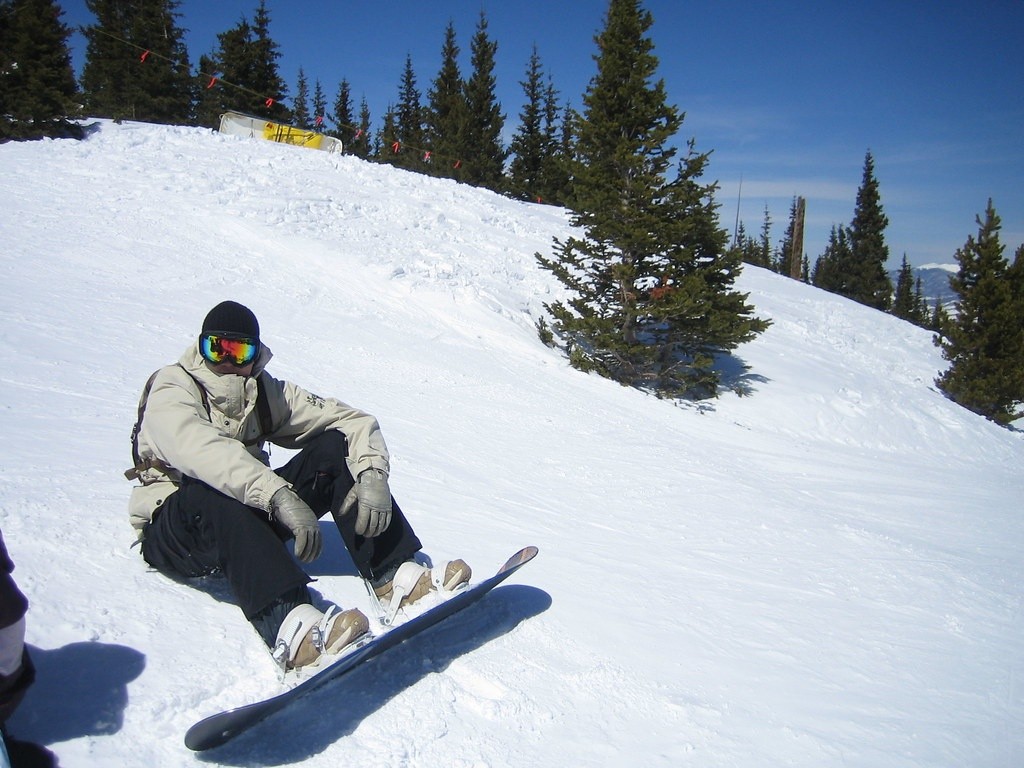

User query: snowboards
[184,545,542,751]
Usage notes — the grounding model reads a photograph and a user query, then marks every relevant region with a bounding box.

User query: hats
[202,301,259,338]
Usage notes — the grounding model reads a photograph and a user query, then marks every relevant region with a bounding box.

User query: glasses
[199,331,258,368]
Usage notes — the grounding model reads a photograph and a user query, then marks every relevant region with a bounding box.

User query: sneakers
[270,603,369,669]
[364,555,471,610]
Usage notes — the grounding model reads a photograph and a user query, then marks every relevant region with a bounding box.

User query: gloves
[338,468,392,539]
[270,485,323,564]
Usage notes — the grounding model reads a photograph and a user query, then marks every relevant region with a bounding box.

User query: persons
[125,301,472,669]
